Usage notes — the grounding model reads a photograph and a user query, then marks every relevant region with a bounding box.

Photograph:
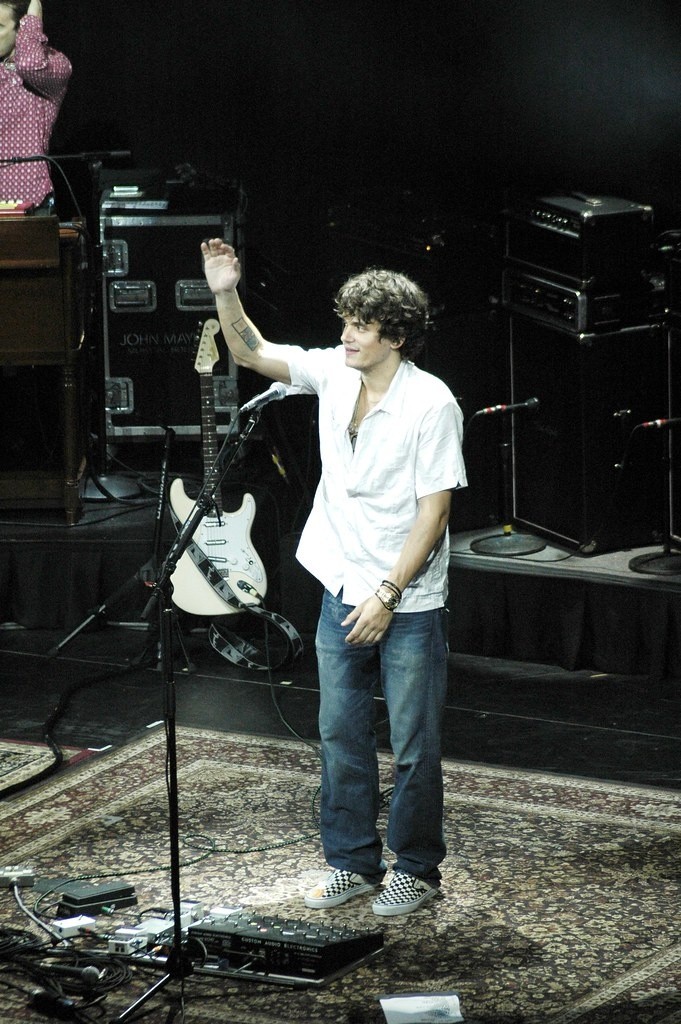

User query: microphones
[477,398,539,415]
[29,986,75,1015]
[643,417,681,427]
[34,962,100,987]
[240,382,287,413]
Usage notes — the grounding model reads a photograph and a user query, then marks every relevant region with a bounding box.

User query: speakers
[504,316,681,556]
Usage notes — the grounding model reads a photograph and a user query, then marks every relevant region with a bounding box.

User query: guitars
[167,320,268,615]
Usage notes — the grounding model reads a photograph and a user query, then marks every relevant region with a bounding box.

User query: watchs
[376,588,399,609]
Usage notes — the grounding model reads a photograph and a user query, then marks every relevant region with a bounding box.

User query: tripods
[25,411,309,1024]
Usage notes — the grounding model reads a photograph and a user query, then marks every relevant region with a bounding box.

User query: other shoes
[372,872,437,917]
[304,869,381,909]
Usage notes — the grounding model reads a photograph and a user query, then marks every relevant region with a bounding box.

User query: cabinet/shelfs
[0,214,89,527]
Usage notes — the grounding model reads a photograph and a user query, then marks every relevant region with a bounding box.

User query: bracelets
[375,580,402,611]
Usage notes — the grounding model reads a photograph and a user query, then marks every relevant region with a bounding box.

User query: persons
[0,0,72,208]
[201,236,468,915]
